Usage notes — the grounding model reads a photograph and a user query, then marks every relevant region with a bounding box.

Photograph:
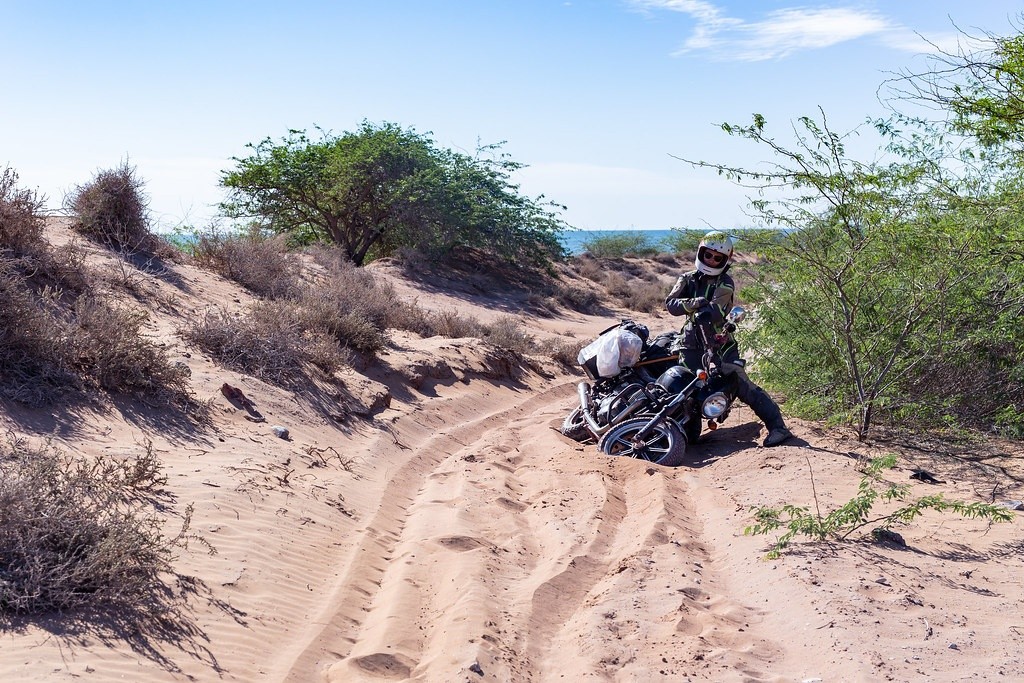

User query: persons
[664,231,791,447]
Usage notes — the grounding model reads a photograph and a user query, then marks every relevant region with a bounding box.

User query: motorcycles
[560,299,745,465]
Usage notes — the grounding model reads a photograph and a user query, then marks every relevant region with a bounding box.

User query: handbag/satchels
[688,297,707,309]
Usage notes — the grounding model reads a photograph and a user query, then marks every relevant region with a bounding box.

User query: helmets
[695,230,733,276]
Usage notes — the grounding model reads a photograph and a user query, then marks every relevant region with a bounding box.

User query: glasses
[705,251,722,262]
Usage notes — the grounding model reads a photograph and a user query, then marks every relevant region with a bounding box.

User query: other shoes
[763,426,791,447]
